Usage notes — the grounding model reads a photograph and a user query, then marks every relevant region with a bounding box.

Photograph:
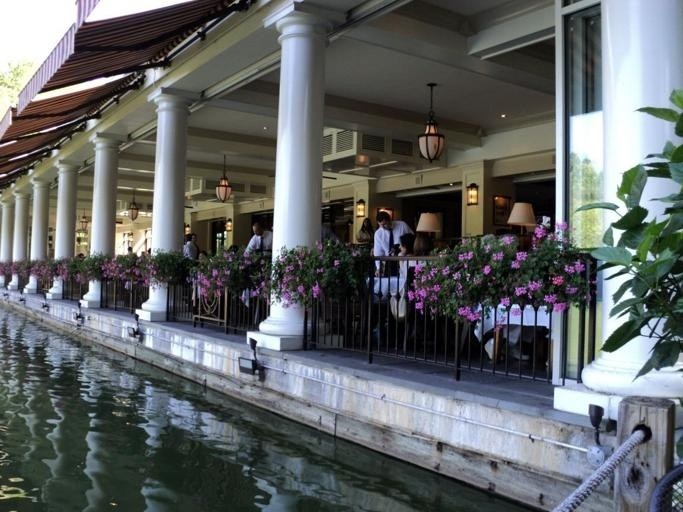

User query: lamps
[356,199,366,217]
[417,77,445,163]
[128,188,139,221]
[1,293,145,344]
[80,206,88,230]
[507,202,537,235]
[225,217,232,232]
[236,337,265,383]
[215,154,231,203]
[184,224,191,235]
[415,209,442,252]
[582,401,618,468]
[466,182,479,207]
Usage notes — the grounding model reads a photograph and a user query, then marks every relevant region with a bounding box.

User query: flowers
[246,236,366,311]
[183,244,263,299]
[403,215,596,331]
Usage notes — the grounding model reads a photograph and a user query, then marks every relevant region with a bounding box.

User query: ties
[387,228,395,255]
[259,237,264,250]
